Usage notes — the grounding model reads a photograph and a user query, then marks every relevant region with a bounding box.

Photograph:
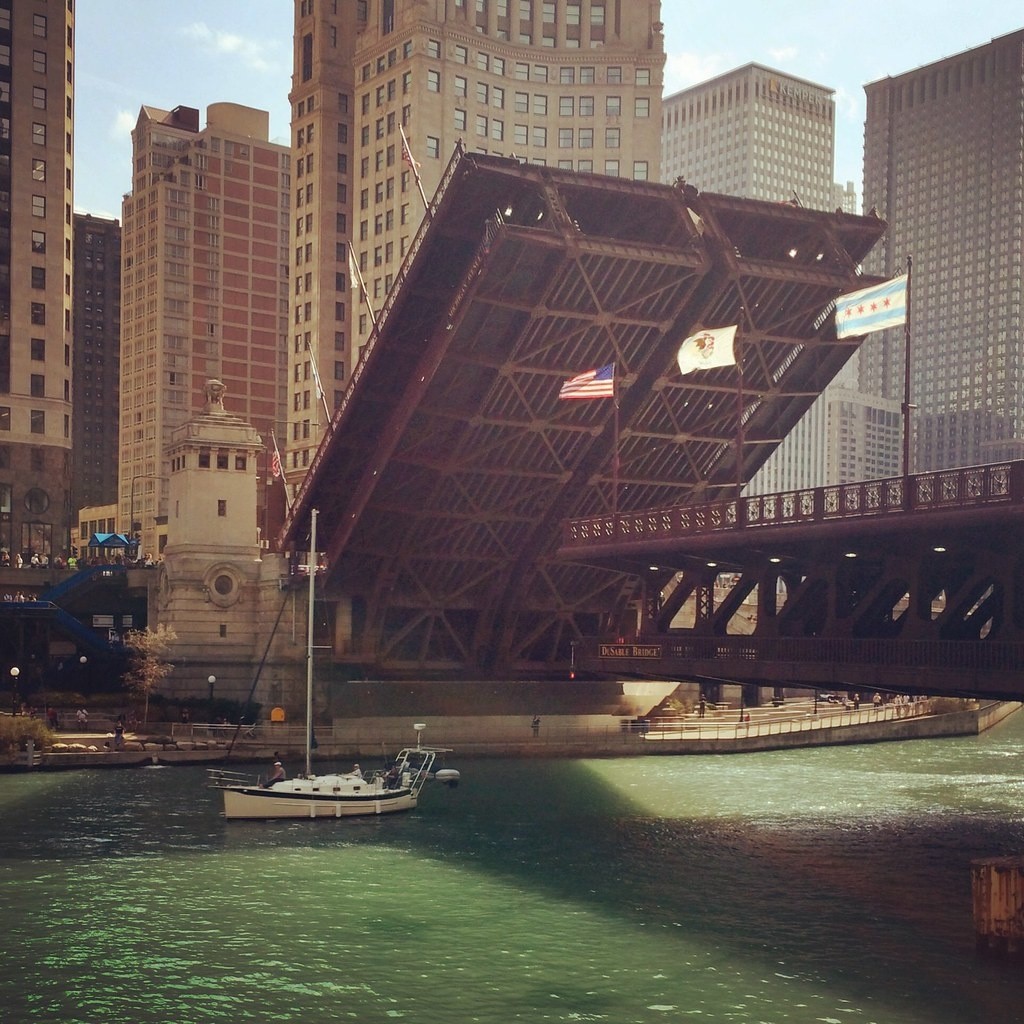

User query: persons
[853,694,860,712]
[349,764,362,780]
[181,707,268,741]
[872,690,929,706]
[533,714,542,737]
[21,702,37,719]
[113,719,127,748]
[47,703,60,731]
[698,693,706,719]
[115,710,138,731]
[381,765,398,790]
[77,705,89,729]
[1,544,166,622]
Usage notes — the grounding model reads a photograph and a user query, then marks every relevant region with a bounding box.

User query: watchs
[258,761,287,789]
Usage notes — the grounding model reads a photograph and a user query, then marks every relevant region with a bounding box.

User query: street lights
[80,656,87,697]
[208,675,216,729]
[10,667,20,716]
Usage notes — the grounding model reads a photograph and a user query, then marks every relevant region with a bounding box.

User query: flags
[557,362,617,399]
[677,322,738,375]
[269,129,415,481]
[834,273,908,340]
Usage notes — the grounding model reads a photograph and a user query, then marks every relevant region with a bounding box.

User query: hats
[274,762,281,766]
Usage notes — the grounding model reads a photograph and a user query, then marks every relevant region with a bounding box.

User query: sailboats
[205,508,435,821]
